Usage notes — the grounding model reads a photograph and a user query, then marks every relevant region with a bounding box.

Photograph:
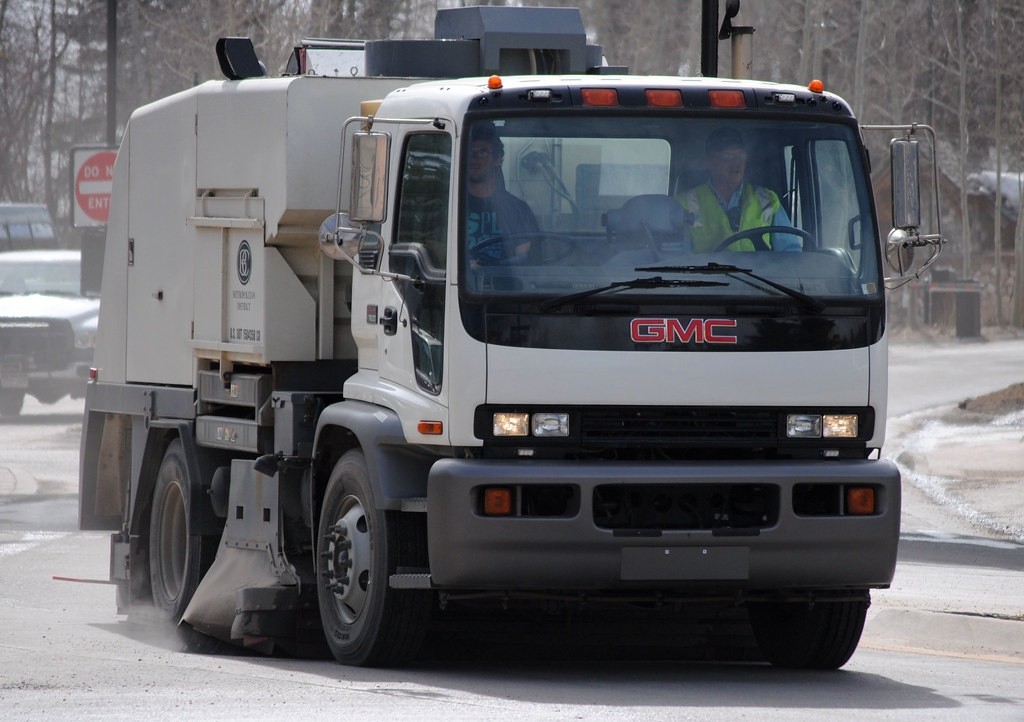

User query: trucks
[77,6,948,670]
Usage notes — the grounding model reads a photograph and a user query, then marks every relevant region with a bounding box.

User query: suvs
[0,249,100,414]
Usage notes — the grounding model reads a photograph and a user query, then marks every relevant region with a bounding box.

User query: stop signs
[69,146,120,230]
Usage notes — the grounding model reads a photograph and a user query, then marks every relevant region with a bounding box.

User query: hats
[706,126,744,152]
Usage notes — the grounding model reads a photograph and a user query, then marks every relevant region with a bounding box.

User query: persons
[666,127,803,256]
[423,130,536,269]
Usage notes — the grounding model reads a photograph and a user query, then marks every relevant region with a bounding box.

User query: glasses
[471,149,494,159]
[712,152,746,162]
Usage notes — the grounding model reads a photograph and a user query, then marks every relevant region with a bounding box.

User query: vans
[0,203,59,253]
